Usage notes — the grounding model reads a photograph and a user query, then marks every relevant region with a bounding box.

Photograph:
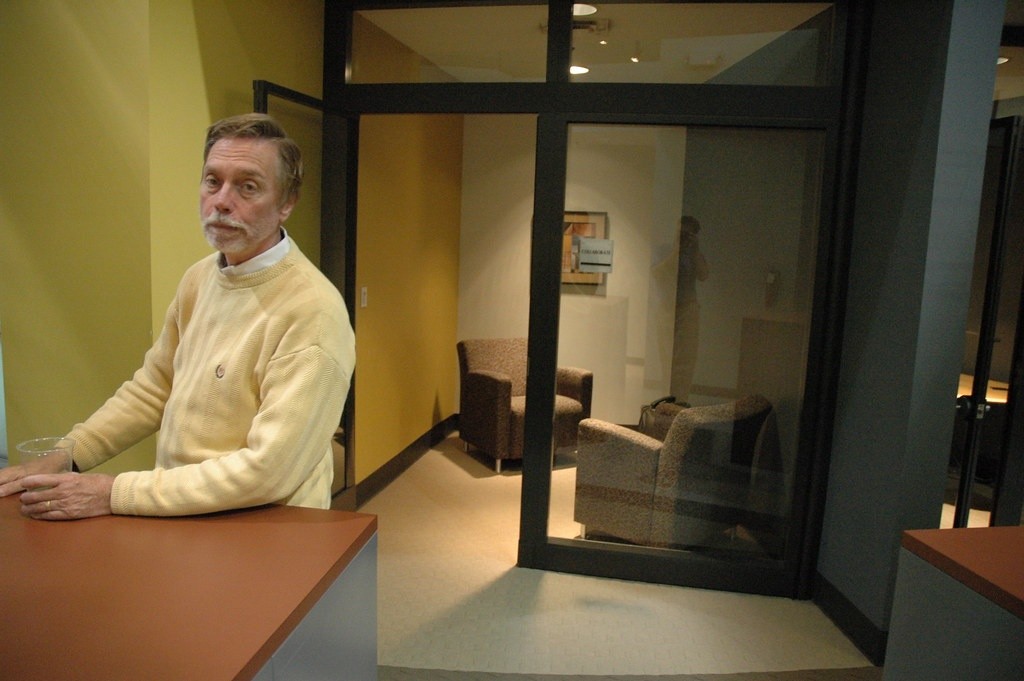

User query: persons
[670,215,709,403]
[0,113,356,520]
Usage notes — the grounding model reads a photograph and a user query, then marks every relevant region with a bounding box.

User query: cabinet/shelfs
[881,526,1024,680]
[0,481,380,681]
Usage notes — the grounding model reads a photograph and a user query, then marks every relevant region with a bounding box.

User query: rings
[46,501,51,507]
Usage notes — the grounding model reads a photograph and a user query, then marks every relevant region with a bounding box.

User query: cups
[15,436,76,492]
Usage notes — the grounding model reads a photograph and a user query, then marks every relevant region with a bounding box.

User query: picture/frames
[561,211,608,287]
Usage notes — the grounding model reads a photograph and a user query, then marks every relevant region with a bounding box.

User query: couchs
[457,338,593,473]
[574,392,775,550]
